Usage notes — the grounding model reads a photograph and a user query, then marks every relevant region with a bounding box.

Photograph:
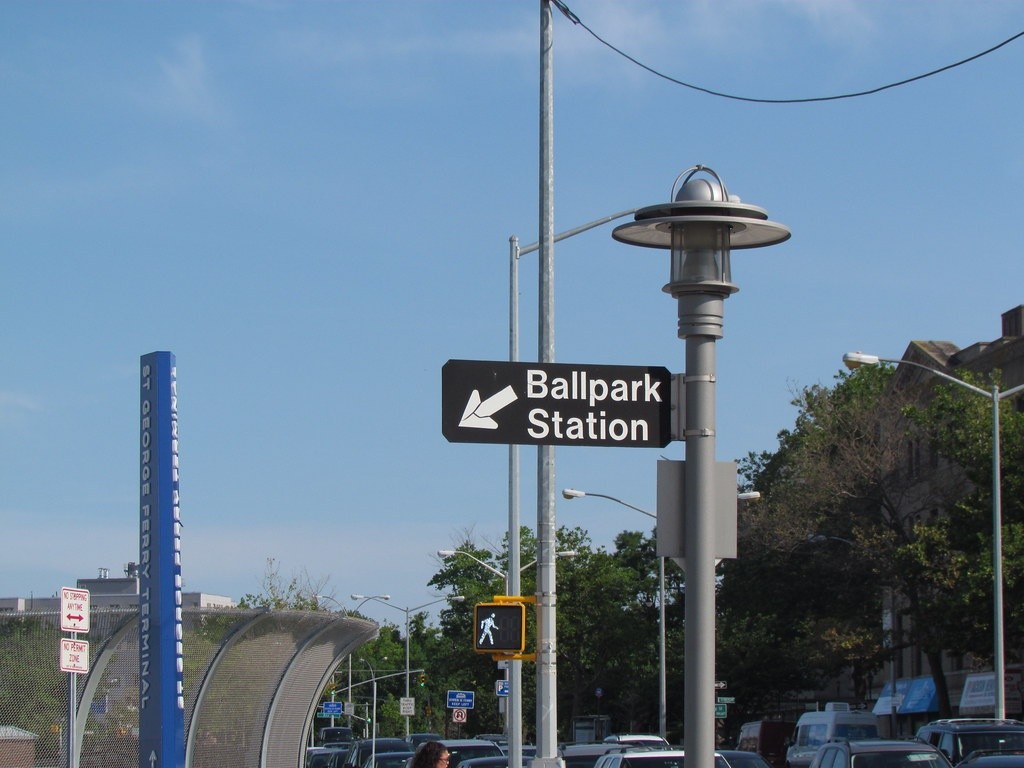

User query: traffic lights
[473,602,525,653]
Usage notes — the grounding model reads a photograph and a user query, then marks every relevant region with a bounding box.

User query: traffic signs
[442,359,674,448]
[714,681,727,690]
[60,586,92,633]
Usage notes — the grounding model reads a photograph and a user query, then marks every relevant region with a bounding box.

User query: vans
[734,720,798,768]
[782,702,882,768]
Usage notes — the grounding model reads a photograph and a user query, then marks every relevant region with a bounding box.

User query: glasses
[439,758,448,763]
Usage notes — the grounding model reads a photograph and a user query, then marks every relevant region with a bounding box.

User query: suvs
[915,718,1024,767]
[808,736,953,768]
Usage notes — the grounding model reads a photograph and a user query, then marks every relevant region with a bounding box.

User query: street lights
[561,488,761,737]
[351,593,465,736]
[316,594,391,727]
[437,547,582,768]
[842,352,1024,718]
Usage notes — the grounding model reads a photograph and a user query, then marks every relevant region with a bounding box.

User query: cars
[556,742,634,768]
[472,734,532,746]
[500,744,564,759]
[455,755,562,768]
[953,749,1024,768]
[305,725,502,768]
[594,750,732,768]
[602,734,674,752]
[715,750,775,768]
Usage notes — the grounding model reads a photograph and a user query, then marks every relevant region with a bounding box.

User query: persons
[410,742,449,768]
